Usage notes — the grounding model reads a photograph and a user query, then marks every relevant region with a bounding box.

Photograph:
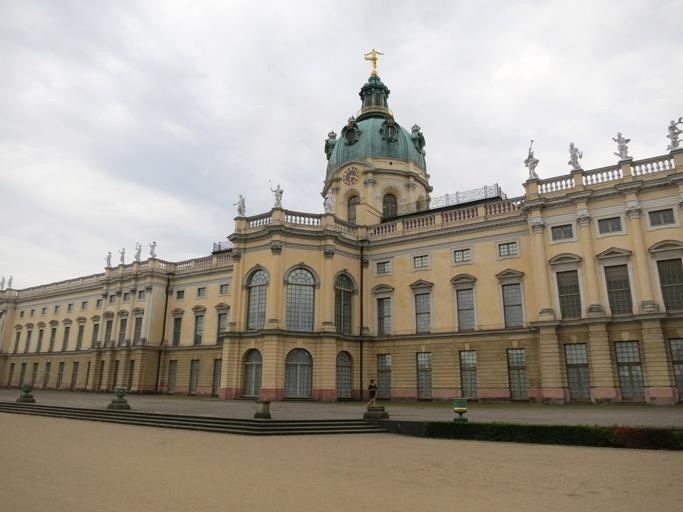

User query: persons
[364,379,377,407]
